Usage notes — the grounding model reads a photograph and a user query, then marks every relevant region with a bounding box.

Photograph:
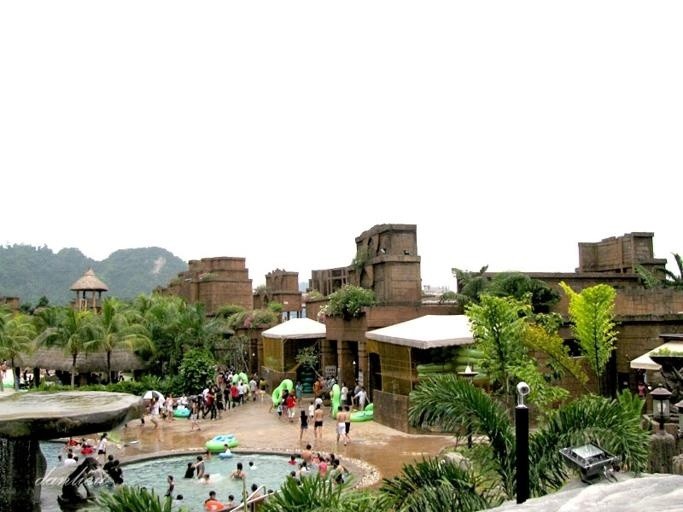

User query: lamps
[648,383,673,429]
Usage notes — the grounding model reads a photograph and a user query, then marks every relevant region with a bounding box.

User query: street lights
[456,361,478,449]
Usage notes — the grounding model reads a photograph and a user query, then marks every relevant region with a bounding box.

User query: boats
[349,403,373,422]
[331,384,340,419]
[229,372,248,402]
[172,408,190,418]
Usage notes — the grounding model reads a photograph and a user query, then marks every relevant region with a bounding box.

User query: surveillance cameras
[517,382,530,395]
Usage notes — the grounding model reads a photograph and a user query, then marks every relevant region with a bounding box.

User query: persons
[164,475,176,496]
[57,431,123,485]
[637,380,645,396]
[138,390,188,429]
[177,494,183,500]
[183,439,344,512]
[0,360,7,379]
[187,363,371,448]
[22,369,124,390]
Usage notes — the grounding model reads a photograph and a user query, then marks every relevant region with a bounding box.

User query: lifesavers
[348,402,374,422]
[218,452,233,459]
[204,501,224,512]
[173,409,190,418]
[231,372,248,384]
[330,383,341,418]
